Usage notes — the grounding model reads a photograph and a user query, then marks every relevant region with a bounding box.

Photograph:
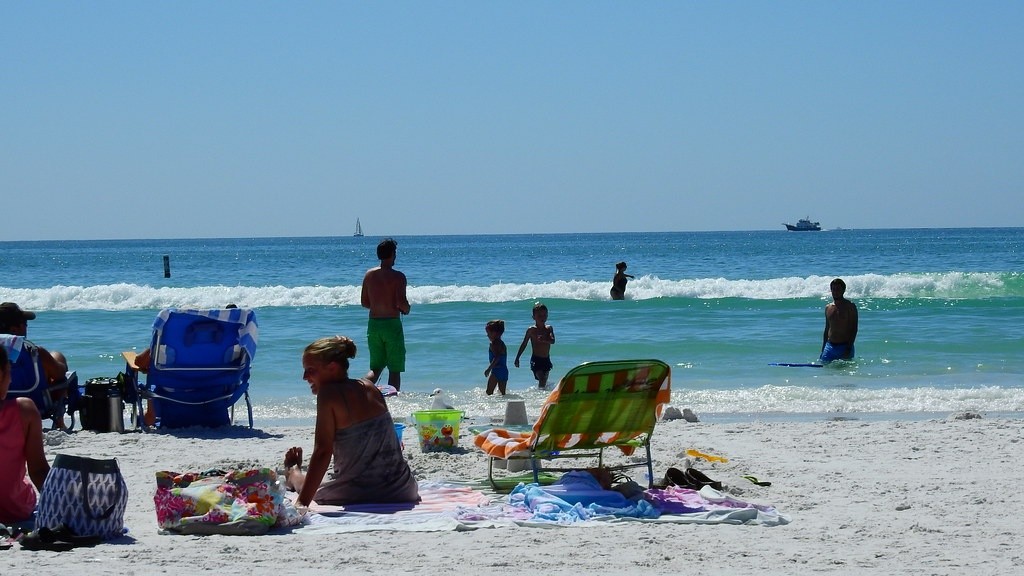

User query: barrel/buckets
[394,422,406,448]
[410,409,467,453]
[84,376,124,435]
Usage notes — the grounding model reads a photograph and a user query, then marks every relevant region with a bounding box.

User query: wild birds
[430,388,469,419]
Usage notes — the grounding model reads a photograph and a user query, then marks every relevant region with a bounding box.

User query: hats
[0,302,35,323]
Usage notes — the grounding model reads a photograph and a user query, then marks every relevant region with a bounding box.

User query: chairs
[468,361,671,492]
[121,307,257,430]
[0,334,78,432]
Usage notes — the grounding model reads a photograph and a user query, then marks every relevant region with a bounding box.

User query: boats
[783,218,821,231]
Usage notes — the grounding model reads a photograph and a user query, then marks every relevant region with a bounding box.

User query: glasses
[12,319,27,323]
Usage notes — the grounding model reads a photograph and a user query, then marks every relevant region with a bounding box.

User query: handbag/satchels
[35,454,128,540]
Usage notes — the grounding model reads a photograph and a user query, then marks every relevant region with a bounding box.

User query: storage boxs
[84,378,123,432]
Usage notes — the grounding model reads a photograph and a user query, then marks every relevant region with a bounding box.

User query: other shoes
[665,467,721,492]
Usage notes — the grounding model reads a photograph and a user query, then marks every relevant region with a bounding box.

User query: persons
[610,261,634,300]
[134,349,156,426]
[514,304,555,388]
[0,302,69,431]
[285,335,418,516]
[360,239,410,392]
[818,279,859,360]
[0,345,54,525]
[483,320,509,394]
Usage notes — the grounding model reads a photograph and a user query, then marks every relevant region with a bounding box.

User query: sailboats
[353,217,365,237]
